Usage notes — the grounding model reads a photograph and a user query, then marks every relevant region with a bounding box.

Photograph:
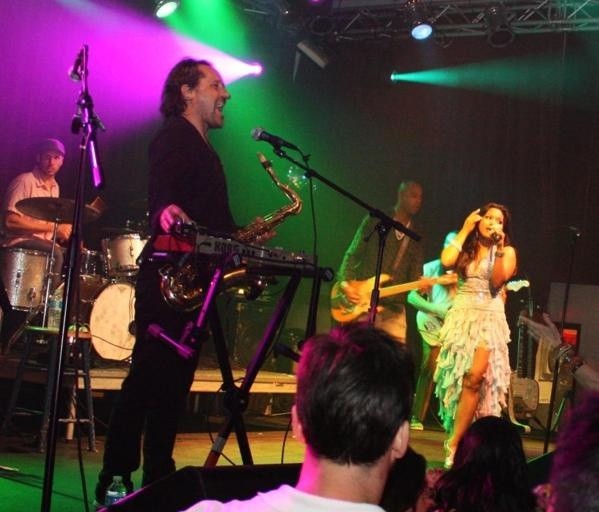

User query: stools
[0,325,98,453]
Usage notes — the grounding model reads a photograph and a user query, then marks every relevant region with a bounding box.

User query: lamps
[406,0,432,40]
[482,5,515,48]
[150,0,180,18]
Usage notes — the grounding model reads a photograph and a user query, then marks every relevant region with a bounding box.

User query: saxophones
[159,152,303,314]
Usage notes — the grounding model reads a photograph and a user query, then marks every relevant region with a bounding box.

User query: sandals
[443,441,455,470]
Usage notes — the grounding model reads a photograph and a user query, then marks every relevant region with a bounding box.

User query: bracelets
[450,239,463,253]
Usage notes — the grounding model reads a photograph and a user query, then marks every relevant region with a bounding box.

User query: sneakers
[410,416,424,431]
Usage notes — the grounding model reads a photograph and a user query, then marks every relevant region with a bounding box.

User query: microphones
[491,233,501,242]
[68,49,84,82]
[251,126,299,150]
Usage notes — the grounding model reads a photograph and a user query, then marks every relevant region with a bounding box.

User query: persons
[3,137,75,296]
[335,177,431,432]
[91,56,277,511]
[181,312,599,512]
[440,202,519,470]
[406,231,464,432]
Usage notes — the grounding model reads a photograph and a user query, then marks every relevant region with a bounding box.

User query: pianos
[153,233,313,264]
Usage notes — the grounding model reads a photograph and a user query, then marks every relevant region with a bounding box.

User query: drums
[0,247,52,314]
[63,249,103,281]
[48,273,135,364]
[102,232,149,279]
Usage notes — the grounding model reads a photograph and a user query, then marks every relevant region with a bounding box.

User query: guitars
[329,269,459,324]
[511,308,539,418]
[416,279,531,347]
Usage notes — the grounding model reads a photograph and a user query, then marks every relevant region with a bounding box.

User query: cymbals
[14,197,101,223]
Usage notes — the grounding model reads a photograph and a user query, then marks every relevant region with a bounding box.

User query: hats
[39,138,65,154]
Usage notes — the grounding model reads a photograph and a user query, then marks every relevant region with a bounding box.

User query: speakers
[95,462,303,512]
